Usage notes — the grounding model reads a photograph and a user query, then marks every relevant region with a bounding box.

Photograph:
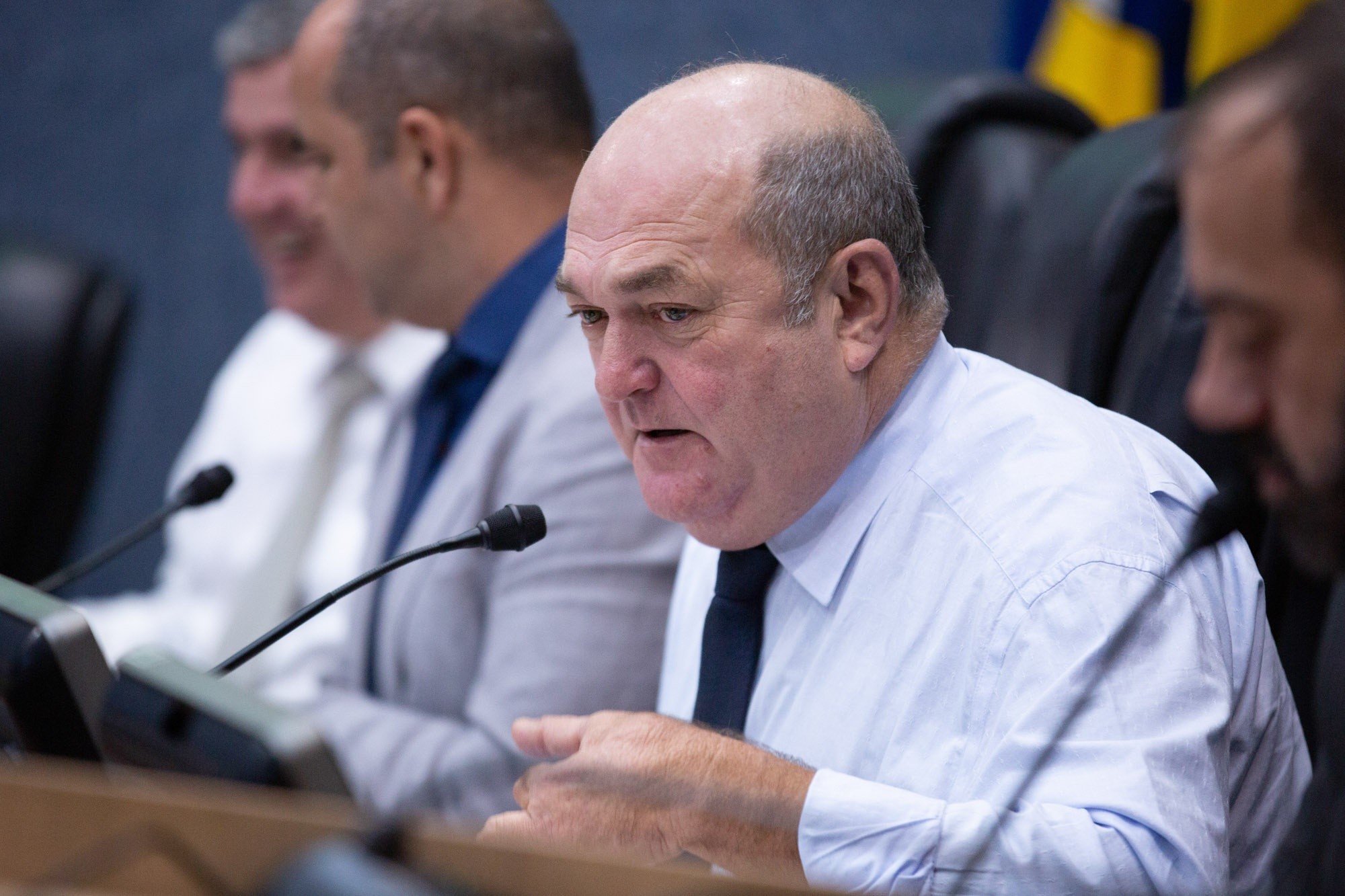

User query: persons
[982,1,1345,896]
[473,61,1314,896]
[55,0,685,896]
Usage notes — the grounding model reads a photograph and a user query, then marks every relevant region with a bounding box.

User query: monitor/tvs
[0,574,352,801]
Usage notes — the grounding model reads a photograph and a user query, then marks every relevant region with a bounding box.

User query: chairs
[0,245,138,595]
[980,107,1257,500]
[870,74,1092,355]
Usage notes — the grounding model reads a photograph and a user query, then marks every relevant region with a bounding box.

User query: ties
[675,540,783,871]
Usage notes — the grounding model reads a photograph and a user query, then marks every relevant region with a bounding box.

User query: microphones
[28,465,236,593]
[945,479,1254,895]
[203,503,547,681]
